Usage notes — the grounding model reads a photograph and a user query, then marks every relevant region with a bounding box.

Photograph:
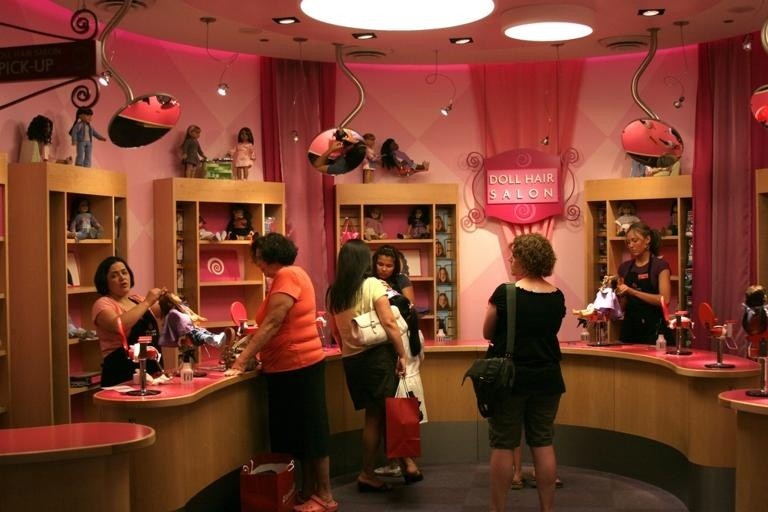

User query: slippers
[293,492,338,512]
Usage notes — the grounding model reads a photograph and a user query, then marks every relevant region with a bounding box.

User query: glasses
[253,256,265,263]
[382,248,395,258]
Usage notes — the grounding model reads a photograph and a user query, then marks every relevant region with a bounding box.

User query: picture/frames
[397,249,421,276]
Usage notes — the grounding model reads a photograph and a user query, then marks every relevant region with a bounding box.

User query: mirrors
[621,28,684,169]
[308,43,365,175]
[94,0,181,148]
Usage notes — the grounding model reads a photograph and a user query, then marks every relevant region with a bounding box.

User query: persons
[323,238,424,494]
[436,293,449,311]
[197,216,227,242]
[361,133,383,184]
[69,197,103,244]
[222,204,259,240]
[90,255,168,388]
[435,214,447,234]
[311,127,367,175]
[68,106,107,169]
[180,124,207,179]
[612,220,672,346]
[223,231,339,512]
[379,138,432,174]
[437,266,451,282]
[372,245,417,310]
[482,232,567,512]
[509,443,565,491]
[362,206,388,241]
[571,275,625,321]
[159,290,226,349]
[433,239,448,259]
[229,126,256,181]
[611,202,643,241]
[24,113,73,166]
[396,206,432,239]
[373,295,428,474]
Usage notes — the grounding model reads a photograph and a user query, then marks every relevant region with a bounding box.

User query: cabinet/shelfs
[336,184,459,345]
[9,163,128,428]
[154,178,285,371]
[584,174,692,341]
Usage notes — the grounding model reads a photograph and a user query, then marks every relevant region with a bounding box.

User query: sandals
[399,457,423,483]
[358,464,395,493]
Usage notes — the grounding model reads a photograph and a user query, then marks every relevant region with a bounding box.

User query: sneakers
[511,476,528,490]
[531,469,563,487]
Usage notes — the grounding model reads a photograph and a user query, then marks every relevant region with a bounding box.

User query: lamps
[98,19,117,88]
[541,43,565,145]
[199,17,239,95]
[674,19,689,108]
[425,49,457,116]
[741,0,765,51]
[501,5,594,42]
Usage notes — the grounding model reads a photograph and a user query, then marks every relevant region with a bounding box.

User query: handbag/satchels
[101,317,137,387]
[462,282,516,418]
[386,374,421,460]
[240,453,297,511]
[351,279,408,346]
